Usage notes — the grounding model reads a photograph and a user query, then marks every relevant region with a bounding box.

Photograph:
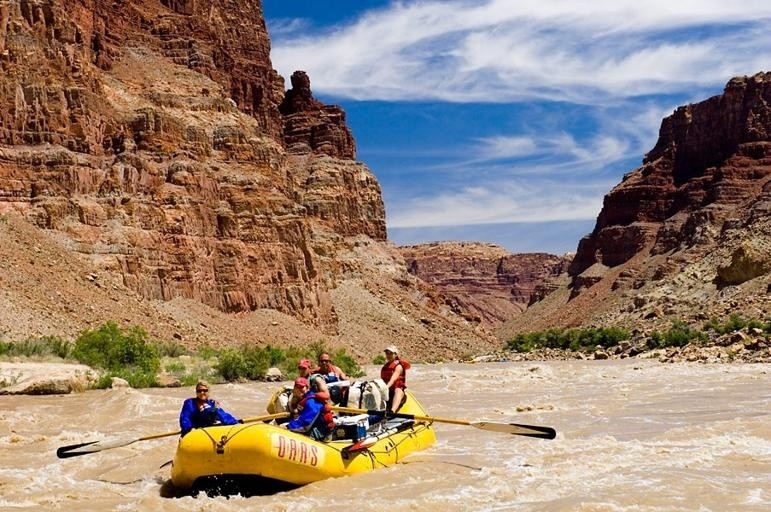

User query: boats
[165,385,442,494]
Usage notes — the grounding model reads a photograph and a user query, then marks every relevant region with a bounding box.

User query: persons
[378,343,407,418]
[312,352,350,412]
[178,381,236,434]
[283,378,334,442]
[267,384,295,425]
[288,357,330,415]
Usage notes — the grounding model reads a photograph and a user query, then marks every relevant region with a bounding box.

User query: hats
[294,377,308,387]
[297,359,312,368]
[383,345,400,355]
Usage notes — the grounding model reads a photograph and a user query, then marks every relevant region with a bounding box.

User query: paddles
[58,412,289,458]
[332,405,555,439]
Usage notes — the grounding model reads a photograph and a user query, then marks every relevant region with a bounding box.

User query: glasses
[197,389,208,393]
